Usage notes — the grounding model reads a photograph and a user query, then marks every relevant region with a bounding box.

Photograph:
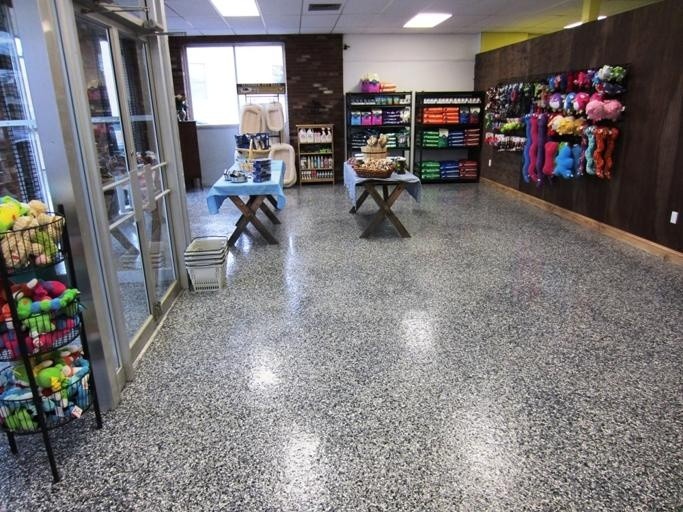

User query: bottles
[302,170,333,180]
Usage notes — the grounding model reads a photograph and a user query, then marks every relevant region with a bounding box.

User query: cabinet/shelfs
[295,124,334,187]
[0,201,102,481]
[345,90,484,184]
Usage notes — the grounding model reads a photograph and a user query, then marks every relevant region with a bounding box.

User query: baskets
[234,139,269,159]
[360,146,387,160]
[353,166,393,179]
[184,235,229,295]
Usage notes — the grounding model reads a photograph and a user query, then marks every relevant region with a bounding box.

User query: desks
[214,159,286,248]
[343,160,419,239]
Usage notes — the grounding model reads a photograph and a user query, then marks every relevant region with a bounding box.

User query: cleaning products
[298,128,333,179]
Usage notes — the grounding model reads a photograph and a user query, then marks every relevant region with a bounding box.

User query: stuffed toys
[481,65,626,189]
[0,193,92,437]
